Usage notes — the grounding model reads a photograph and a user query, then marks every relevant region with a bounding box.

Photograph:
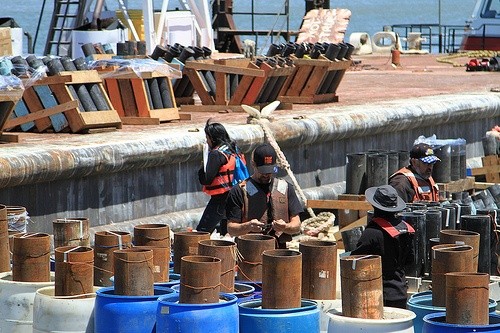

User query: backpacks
[230,156,251,188]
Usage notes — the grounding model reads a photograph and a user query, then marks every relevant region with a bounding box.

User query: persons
[350,184,415,309]
[224,144,304,249]
[388,143,442,203]
[195,119,247,237]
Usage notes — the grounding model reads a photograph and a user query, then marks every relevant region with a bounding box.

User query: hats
[364,184,408,215]
[249,142,280,176]
[408,142,442,165]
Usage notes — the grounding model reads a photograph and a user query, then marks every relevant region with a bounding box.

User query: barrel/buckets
[0,259,500,333]
[115,9,144,42]
